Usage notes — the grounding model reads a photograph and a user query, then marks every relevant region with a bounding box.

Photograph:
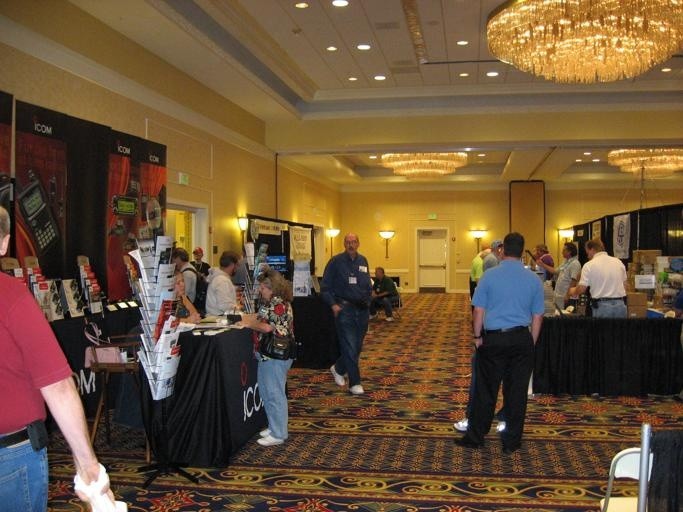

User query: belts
[339,300,365,309]
[596,298,623,301]
[486,327,529,334]
[0,427,30,448]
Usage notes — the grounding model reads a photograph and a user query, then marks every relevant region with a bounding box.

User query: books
[4,234,182,401]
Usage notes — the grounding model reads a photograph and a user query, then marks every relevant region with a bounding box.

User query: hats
[192,247,203,254]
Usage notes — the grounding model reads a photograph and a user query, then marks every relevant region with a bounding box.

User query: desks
[291,288,341,369]
[150,317,267,469]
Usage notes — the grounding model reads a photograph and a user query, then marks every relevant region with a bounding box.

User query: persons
[203,250,244,315]
[318,232,372,395]
[453,237,631,434]
[188,251,211,316]
[368,266,399,321]
[232,269,294,446]
[169,269,200,326]
[0,205,127,512]
[171,248,206,324]
[453,233,545,455]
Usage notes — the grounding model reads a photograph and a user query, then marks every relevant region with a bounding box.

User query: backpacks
[180,268,208,313]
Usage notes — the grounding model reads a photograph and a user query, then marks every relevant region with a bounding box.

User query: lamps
[559,230,573,242]
[470,230,488,254]
[327,230,340,257]
[381,152,467,175]
[486,0,683,84]
[608,146,683,175]
[238,218,248,251]
[379,232,394,258]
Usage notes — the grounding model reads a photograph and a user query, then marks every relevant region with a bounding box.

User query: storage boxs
[626,250,662,318]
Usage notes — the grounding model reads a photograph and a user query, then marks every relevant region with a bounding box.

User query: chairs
[600,447,653,512]
[84,322,151,464]
[376,287,402,320]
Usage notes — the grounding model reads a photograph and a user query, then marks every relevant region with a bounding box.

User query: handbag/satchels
[84,321,125,368]
[258,331,297,360]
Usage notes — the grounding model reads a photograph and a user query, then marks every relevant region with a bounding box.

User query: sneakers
[453,418,521,454]
[330,364,346,387]
[257,428,289,446]
[349,384,365,395]
